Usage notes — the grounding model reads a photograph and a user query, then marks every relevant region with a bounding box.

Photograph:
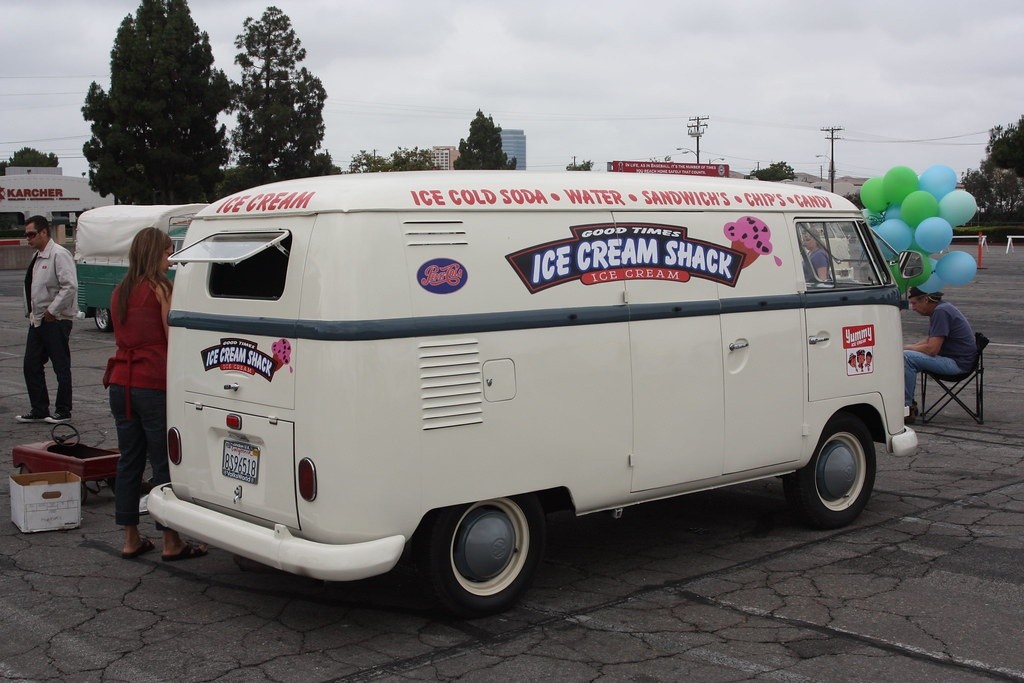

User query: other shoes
[904,408,915,423]
[912,404,919,416]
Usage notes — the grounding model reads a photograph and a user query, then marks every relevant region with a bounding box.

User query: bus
[146,170,933,621]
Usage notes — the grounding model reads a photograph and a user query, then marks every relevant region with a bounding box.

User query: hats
[908,287,928,299]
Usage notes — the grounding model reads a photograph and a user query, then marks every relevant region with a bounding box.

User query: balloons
[857,165,978,296]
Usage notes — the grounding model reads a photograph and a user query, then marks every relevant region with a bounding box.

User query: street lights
[676,148,699,164]
[816,155,834,193]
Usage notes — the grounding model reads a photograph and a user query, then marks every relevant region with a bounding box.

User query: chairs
[920,332,989,426]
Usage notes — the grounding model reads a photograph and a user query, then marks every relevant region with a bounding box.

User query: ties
[24,252,39,313]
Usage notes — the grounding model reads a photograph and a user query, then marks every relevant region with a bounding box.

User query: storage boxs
[9,470,81,533]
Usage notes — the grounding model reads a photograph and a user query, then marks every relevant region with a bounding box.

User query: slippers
[162,541,209,560]
[122,538,155,559]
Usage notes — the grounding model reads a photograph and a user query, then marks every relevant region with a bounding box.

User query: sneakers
[15,410,45,422]
[44,412,72,423]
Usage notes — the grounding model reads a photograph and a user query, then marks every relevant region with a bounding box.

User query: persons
[14,215,79,425]
[799,228,828,282]
[903,286,978,422]
[102,227,210,559]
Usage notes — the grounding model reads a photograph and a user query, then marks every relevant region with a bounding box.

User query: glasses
[24,231,38,238]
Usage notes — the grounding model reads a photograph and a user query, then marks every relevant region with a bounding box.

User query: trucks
[74,204,210,334]
